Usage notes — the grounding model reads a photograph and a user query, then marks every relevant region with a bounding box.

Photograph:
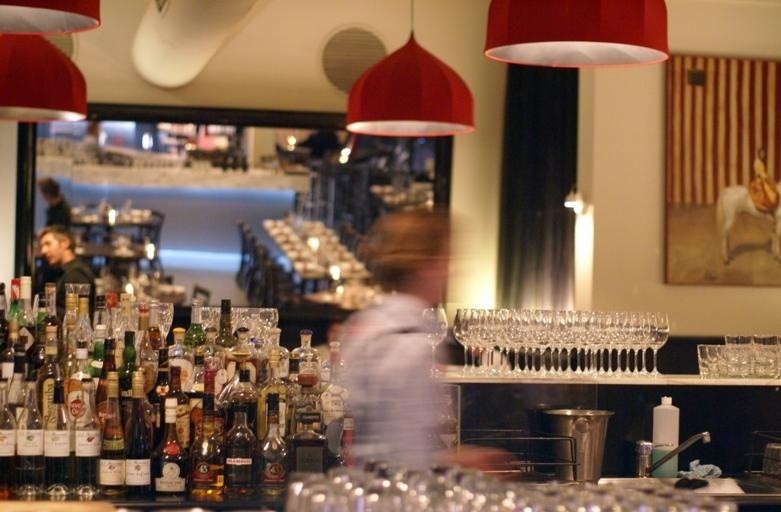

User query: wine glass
[453,307,670,378]
[418,304,448,383]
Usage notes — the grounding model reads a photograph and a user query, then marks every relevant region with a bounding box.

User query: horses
[714,181,781,267]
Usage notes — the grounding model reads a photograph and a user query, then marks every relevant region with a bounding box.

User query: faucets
[632,430,711,478]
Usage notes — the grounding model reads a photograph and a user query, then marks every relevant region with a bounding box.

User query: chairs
[234,216,387,313]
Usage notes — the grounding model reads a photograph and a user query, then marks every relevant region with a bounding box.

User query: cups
[694,333,779,377]
[284,463,735,512]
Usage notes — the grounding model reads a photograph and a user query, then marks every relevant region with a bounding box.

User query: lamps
[484,1,672,69]
[346,0,477,140]
[1,1,104,35]
[0,34,90,123]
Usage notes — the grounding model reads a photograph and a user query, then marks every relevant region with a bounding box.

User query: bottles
[1,276,357,510]
[762,441,781,476]
[635,437,654,478]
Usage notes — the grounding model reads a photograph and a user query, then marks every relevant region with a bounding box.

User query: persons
[39,227,98,333]
[38,176,76,227]
[753,147,781,204]
[327,208,521,480]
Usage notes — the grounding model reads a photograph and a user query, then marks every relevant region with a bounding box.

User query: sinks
[597,476,781,505]
